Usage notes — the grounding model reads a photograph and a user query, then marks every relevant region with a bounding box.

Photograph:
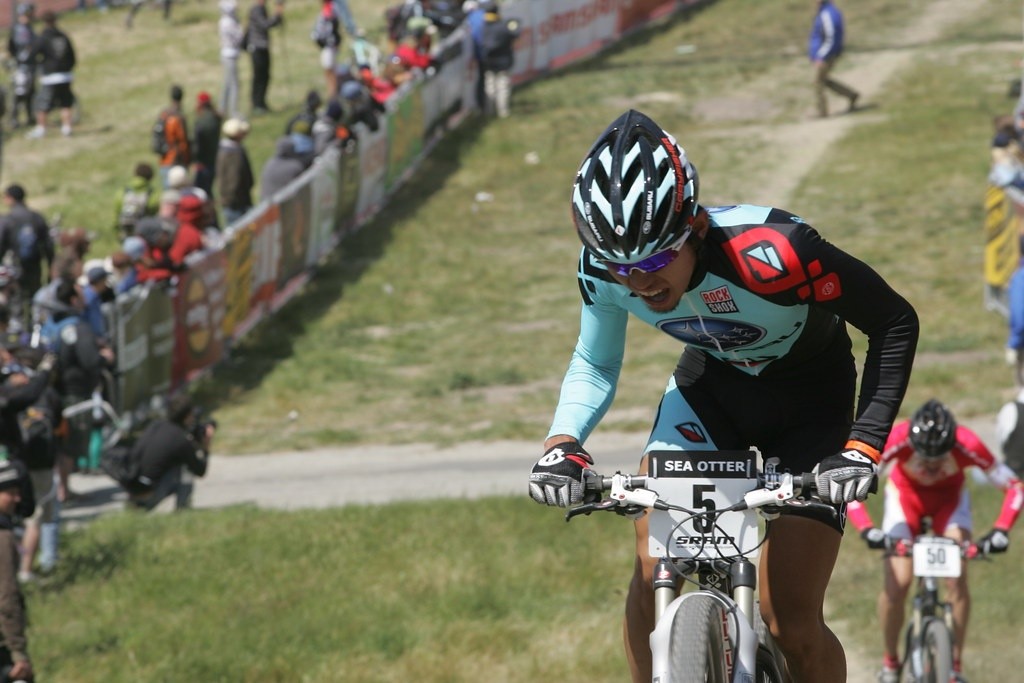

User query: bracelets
[845,440,884,465]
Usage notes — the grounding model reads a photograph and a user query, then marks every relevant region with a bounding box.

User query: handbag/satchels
[7,212,42,259]
[99,434,141,484]
[151,109,177,154]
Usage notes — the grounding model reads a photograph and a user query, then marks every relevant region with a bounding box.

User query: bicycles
[878,522,997,683]
[564,451,880,683]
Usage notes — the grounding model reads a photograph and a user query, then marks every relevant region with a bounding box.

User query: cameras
[191,407,216,442]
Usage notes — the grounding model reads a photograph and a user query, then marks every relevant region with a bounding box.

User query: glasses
[594,245,682,278]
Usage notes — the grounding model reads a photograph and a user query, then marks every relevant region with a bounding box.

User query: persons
[808,0,859,119]
[0,0,523,232]
[0,162,221,587]
[528,110,919,683]
[982,78,1024,483]
[846,400,1024,683]
[0,458,36,683]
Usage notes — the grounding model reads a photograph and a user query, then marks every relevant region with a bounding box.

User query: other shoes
[877,664,902,683]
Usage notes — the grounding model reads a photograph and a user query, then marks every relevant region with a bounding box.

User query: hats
[0,445,21,490]
[326,99,342,114]
[221,118,250,138]
[177,193,204,223]
[342,81,359,99]
[15,2,33,14]
[165,164,186,187]
[88,267,112,282]
[199,91,210,107]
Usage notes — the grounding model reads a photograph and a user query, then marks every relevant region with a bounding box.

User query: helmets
[571,109,700,265]
[907,397,957,458]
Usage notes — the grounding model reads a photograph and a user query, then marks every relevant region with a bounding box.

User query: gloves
[812,447,878,504]
[859,527,892,552]
[528,442,602,517]
[977,529,1009,555]
